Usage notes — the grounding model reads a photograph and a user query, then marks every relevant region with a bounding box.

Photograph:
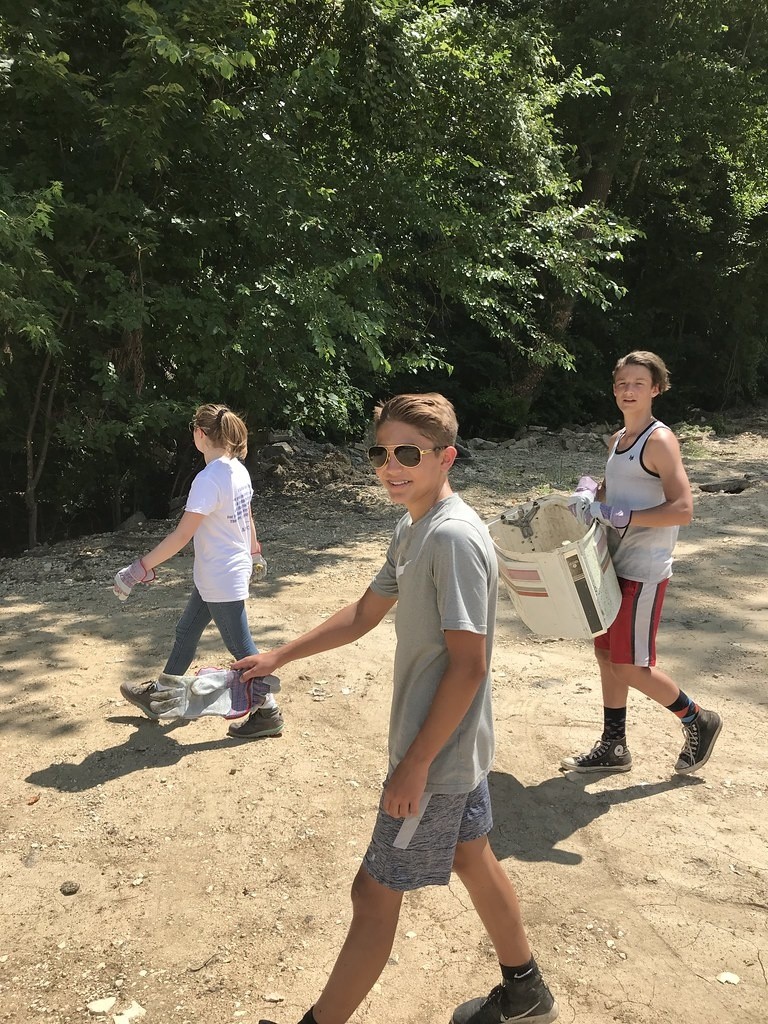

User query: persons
[112,405,285,738]
[561,349,723,774]
[230,393,559,1024]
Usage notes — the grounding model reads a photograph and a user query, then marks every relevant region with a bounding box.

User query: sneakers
[259,1019,278,1024]
[120,680,162,720]
[561,734,633,772]
[451,981,559,1024]
[229,706,284,738]
[675,706,723,774]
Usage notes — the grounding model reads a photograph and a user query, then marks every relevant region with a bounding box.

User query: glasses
[189,422,207,436]
[367,444,448,468]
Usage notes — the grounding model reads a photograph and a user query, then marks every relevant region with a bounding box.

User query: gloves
[251,543,268,581]
[568,476,598,526]
[197,666,282,712]
[113,559,155,600]
[581,501,632,539]
[150,672,254,720]
